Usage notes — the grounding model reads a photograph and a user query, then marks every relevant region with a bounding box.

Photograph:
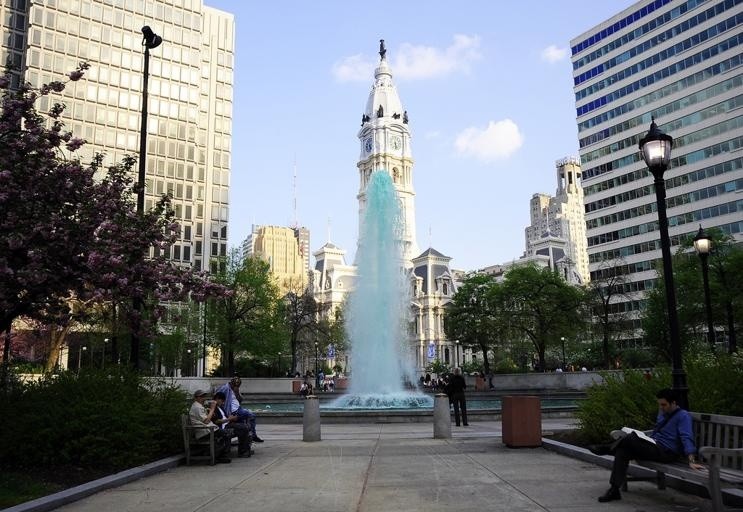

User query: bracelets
[688,459,694,464]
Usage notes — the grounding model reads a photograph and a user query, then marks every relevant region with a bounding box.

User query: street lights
[127,25,163,397]
[102,337,112,344]
[76,344,88,378]
[560,336,565,372]
[276,349,282,378]
[691,223,715,349]
[634,111,691,414]
[313,339,319,388]
[455,340,461,367]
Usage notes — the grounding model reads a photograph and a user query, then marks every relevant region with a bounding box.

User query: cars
[462,370,479,376]
[1,355,48,374]
[282,367,302,378]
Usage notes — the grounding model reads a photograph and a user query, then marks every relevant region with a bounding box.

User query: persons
[188,390,232,464]
[419,368,494,394]
[206,392,251,458]
[280,365,334,396]
[447,368,469,427]
[555,363,588,372]
[214,376,264,444]
[597,387,704,502]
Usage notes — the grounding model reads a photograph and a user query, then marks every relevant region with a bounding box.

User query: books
[621,426,656,445]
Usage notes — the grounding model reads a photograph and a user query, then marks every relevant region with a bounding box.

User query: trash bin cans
[476,378,484,391]
[502,396,542,448]
[293,381,301,392]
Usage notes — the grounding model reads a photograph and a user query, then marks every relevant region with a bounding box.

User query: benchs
[182,413,219,466]
[610,410,743,512]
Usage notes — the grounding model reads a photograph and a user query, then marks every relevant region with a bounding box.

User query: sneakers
[599,488,621,502]
[251,436,264,443]
[589,445,616,456]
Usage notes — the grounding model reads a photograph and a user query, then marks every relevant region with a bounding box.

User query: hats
[194,390,208,396]
